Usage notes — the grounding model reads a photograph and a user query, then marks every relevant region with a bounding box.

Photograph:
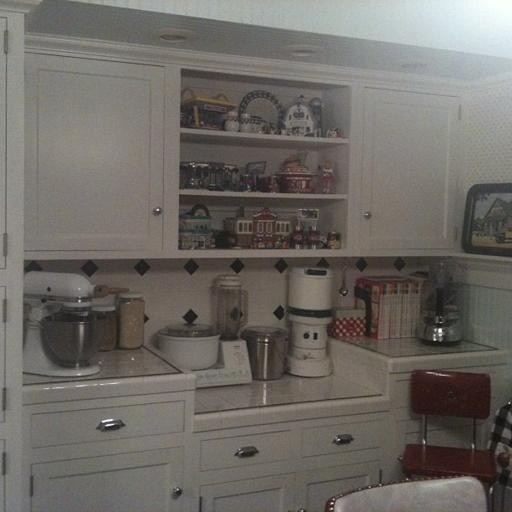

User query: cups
[245,324,288,381]
[214,272,249,339]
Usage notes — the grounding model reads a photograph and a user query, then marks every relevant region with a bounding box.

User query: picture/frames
[459,181,511,262]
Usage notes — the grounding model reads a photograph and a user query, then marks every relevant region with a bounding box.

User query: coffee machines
[287,266,334,377]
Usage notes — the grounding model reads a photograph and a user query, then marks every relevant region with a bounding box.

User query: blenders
[22,271,102,377]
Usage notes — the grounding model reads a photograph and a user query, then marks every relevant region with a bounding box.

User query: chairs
[321,469,492,512]
[400,364,499,491]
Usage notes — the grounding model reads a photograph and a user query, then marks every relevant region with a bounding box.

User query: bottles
[116,292,145,350]
[251,231,342,249]
[179,159,256,193]
[290,215,319,250]
[90,304,117,352]
[224,110,264,134]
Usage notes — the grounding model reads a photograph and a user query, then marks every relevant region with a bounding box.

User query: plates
[190,203,210,216]
[239,90,284,134]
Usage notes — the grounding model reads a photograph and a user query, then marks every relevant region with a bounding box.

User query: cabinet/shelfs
[325,341,510,481]
[192,408,392,512]
[27,385,194,511]
[358,80,461,254]
[173,47,355,253]
[21,47,171,260]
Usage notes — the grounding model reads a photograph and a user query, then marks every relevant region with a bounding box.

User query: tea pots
[415,287,463,345]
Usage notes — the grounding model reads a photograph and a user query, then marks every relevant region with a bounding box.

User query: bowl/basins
[155,334,223,372]
[274,172,317,193]
